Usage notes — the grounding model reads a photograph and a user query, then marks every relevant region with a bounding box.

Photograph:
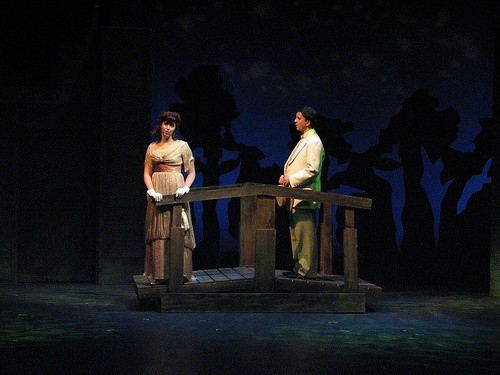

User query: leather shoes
[288,273,317,280]
[282,271,295,276]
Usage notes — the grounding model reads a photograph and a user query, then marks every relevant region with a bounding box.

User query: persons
[142,112,195,282]
[278,104,325,280]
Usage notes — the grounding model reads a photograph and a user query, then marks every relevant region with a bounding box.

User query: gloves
[175,186,190,198]
[147,188,163,202]
